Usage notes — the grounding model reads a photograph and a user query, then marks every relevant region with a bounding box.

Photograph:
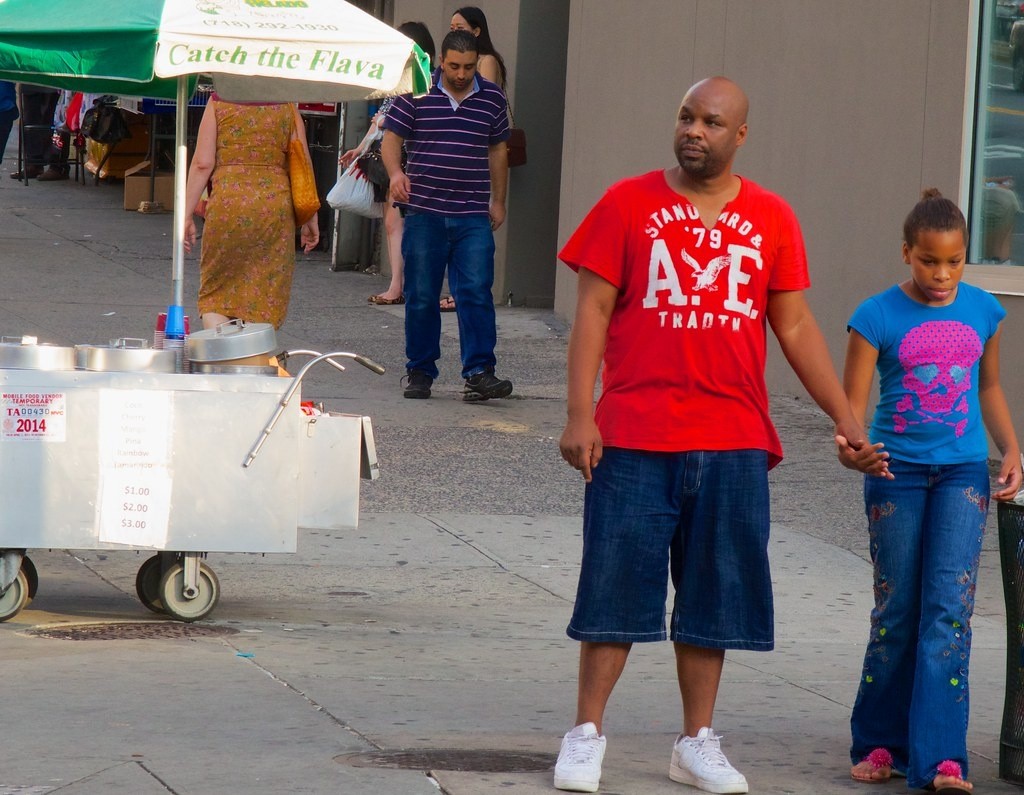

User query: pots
[75,337,176,374]
[184,319,278,375]
[0,335,77,371]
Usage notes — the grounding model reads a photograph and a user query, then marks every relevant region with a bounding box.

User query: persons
[10,83,58,179]
[36,131,71,181]
[554,77,893,793]
[0,80,17,163]
[338,18,456,312]
[184,89,319,331]
[381,30,513,402]
[834,186,1024,795]
[439,6,508,311]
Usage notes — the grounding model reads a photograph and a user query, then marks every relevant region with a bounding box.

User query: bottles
[153,306,192,373]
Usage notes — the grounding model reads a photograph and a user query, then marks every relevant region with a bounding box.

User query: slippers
[440,297,456,312]
[850,748,892,784]
[932,760,972,795]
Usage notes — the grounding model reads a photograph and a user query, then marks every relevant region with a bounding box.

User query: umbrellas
[0,0,432,336]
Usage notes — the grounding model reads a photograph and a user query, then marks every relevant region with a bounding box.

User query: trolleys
[0,350,388,624]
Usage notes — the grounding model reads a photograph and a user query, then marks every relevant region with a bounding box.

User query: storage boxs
[123,161,176,212]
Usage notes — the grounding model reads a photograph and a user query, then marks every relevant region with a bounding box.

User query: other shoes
[368,293,381,302]
[377,295,403,305]
[37,166,70,181]
[9,168,36,178]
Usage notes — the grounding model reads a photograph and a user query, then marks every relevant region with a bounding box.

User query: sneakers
[669,726,748,794]
[461,367,513,401]
[553,722,607,792]
[400,370,433,400]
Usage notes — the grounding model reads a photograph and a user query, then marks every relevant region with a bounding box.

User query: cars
[1006,19,1024,95]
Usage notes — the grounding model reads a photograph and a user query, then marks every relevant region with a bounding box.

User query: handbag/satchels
[356,136,406,187]
[326,118,384,219]
[505,128,528,168]
[287,137,320,227]
